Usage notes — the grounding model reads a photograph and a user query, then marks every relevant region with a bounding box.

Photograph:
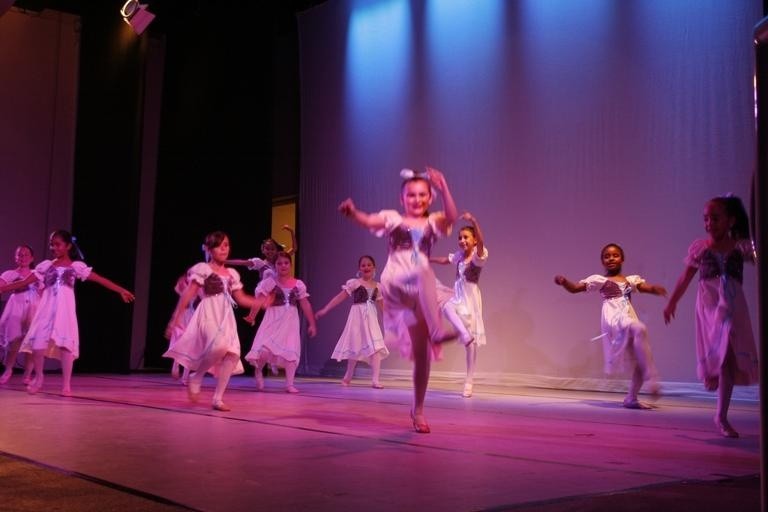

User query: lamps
[118,0,159,34]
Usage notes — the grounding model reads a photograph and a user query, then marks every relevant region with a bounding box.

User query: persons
[555,244,668,409]
[662,193,755,436]
[336,161,457,433]
[0,244,41,384]
[316,254,389,389]
[0,229,135,397]
[428,211,493,399]
[166,224,318,410]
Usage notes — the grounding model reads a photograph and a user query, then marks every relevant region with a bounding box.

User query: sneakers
[188,385,200,401]
[62,389,70,397]
[644,380,659,392]
[624,397,651,409]
[435,333,456,343]
[212,400,230,411]
[342,379,350,385]
[286,385,298,393]
[462,389,472,397]
[27,381,41,393]
[372,383,383,388]
[718,421,739,438]
[24,378,32,384]
[257,379,265,389]
[464,337,474,346]
[704,376,719,391]
[410,410,430,432]
[173,368,180,379]
